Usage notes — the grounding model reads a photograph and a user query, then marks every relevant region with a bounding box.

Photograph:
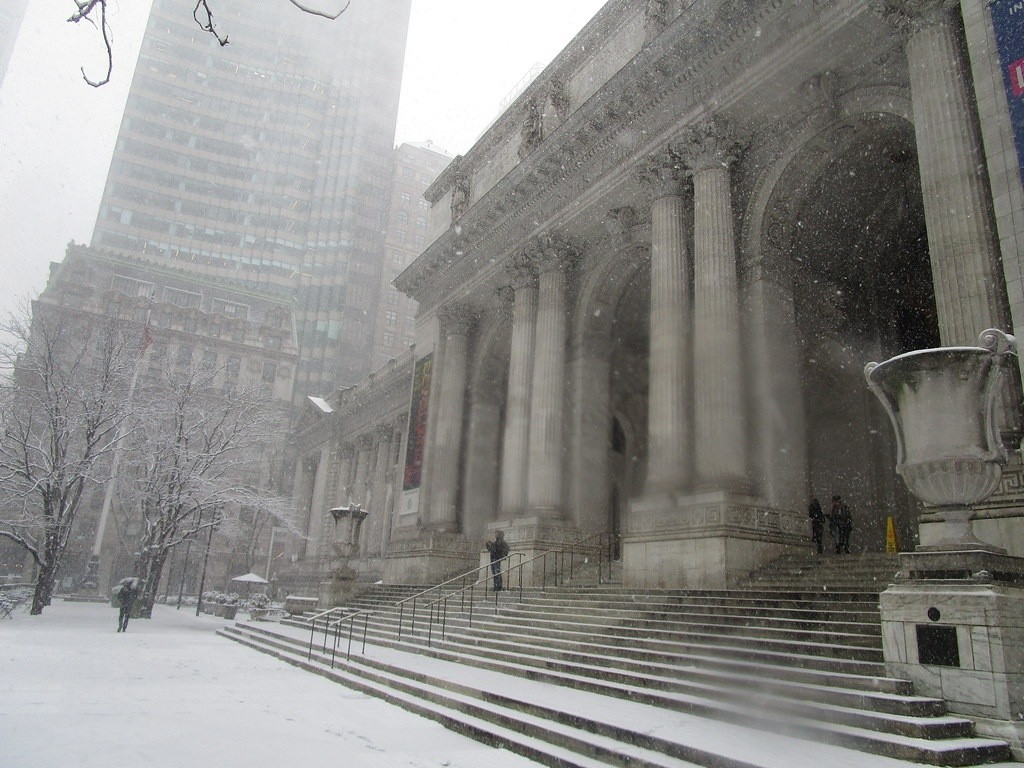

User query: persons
[114,580,136,632]
[830,496,853,553]
[808,498,825,555]
[486,530,508,591]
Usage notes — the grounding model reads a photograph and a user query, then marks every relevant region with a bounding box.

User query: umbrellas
[232,572,270,613]
[121,577,145,586]
